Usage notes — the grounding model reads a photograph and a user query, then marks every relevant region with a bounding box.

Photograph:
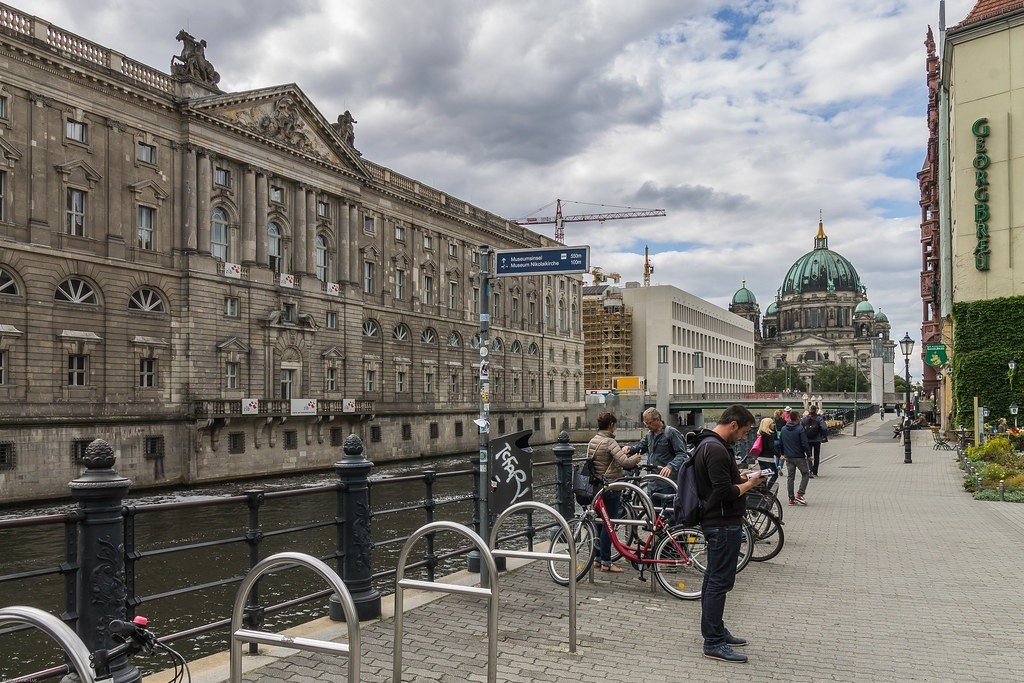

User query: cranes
[505,198,666,245]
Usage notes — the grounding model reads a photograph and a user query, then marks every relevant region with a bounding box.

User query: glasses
[643,418,658,425]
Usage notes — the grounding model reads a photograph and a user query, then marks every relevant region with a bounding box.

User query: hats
[784,406,792,411]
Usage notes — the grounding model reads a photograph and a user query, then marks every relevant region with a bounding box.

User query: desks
[950,429,974,450]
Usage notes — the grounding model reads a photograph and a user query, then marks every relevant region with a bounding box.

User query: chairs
[931,428,951,451]
[892,416,905,438]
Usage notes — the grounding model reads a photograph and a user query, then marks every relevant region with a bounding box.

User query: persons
[688,404,766,663]
[780,411,814,504]
[910,402,914,411]
[344,110,357,144]
[186,35,207,75]
[879,406,885,420]
[783,388,801,399]
[802,406,827,477]
[588,412,641,573]
[624,407,687,557]
[998,418,1009,433]
[757,418,784,489]
[896,401,901,417]
[915,413,928,426]
[773,405,793,474]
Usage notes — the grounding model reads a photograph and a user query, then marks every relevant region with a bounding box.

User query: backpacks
[672,437,731,527]
[805,416,820,439]
[572,437,616,505]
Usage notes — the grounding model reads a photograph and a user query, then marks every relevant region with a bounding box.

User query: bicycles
[546,426,786,601]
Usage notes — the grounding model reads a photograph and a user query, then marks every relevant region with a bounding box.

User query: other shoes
[809,470,813,478]
[795,495,806,505]
[778,466,783,476]
[789,499,797,506]
[813,474,818,478]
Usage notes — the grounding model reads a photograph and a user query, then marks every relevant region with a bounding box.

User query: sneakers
[702,641,748,664]
[723,629,747,646]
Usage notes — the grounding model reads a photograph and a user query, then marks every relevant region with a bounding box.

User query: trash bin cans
[489,428,534,514]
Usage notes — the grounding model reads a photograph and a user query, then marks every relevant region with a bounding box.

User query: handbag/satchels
[749,433,762,457]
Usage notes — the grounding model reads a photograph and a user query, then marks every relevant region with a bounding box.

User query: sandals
[593,560,623,573]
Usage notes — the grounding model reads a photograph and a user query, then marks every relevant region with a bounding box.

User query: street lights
[898,332,917,463]
[843,355,858,436]
